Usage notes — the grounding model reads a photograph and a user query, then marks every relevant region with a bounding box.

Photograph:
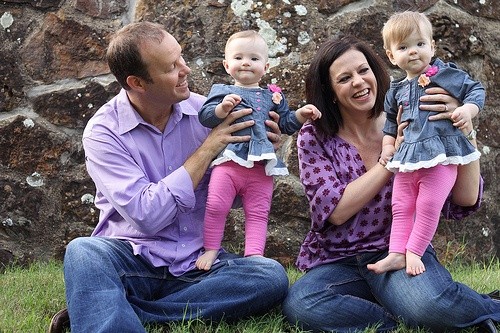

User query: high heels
[487,290,500,301]
[479,318,499,333]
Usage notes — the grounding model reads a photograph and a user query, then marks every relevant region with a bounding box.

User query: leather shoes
[48,308,70,333]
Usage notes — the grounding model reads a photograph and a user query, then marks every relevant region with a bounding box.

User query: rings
[445,105,449,111]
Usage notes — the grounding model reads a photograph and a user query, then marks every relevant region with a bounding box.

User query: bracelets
[466,130,476,140]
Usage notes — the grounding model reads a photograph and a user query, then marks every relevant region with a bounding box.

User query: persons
[194,30,323,270]
[367,10,484,276]
[49,22,289,333]
[282,34,500,333]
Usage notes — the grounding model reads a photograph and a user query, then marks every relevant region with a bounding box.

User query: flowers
[269,84,283,104]
[418,65,438,88]
